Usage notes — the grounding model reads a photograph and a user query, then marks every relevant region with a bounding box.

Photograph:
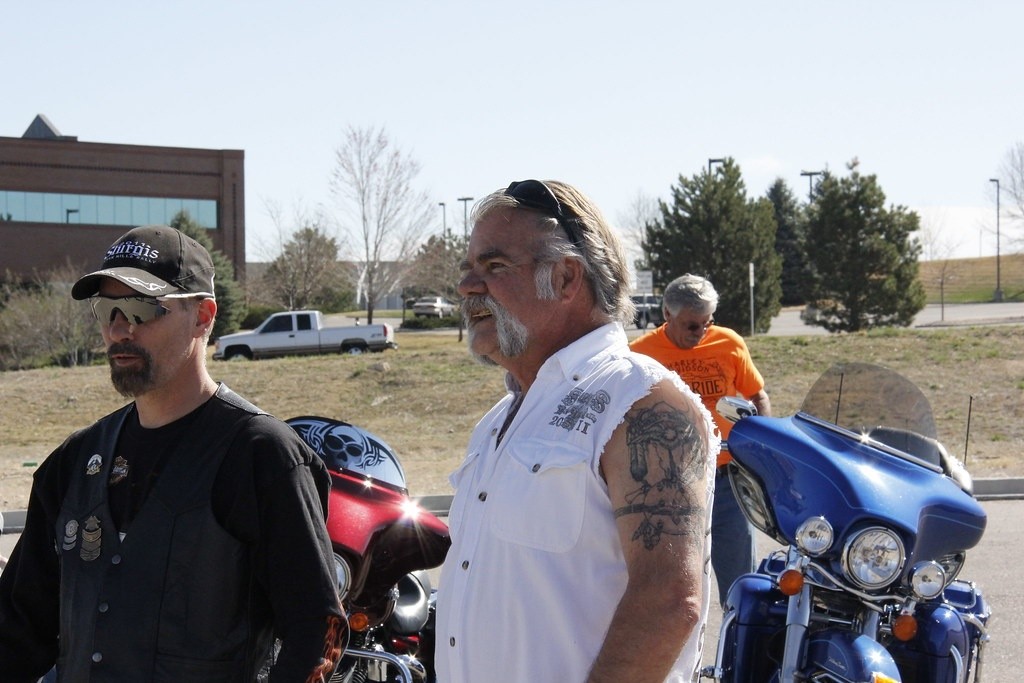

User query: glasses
[687,314,715,331]
[505,179,581,249]
[89,295,173,325]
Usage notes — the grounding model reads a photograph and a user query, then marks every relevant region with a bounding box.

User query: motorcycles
[278,414,454,683]
[703,357,989,683]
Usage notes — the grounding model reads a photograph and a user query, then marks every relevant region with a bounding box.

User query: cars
[413,296,456,319]
[404,298,416,309]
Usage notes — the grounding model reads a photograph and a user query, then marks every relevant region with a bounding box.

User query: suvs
[630,294,666,330]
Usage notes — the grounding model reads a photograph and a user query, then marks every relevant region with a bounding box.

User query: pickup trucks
[211,310,399,361]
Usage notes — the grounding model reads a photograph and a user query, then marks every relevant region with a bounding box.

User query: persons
[434,180,721,683]
[0,225,351,683]
[626,275,771,605]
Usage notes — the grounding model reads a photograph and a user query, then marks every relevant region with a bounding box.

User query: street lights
[66,208,79,224]
[708,158,726,176]
[800,170,822,205]
[458,197,474,243]
[439,202,446,246]
[987,177,1006,301]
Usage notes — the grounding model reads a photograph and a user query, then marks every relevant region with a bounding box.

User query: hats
[71,226,216,300]
